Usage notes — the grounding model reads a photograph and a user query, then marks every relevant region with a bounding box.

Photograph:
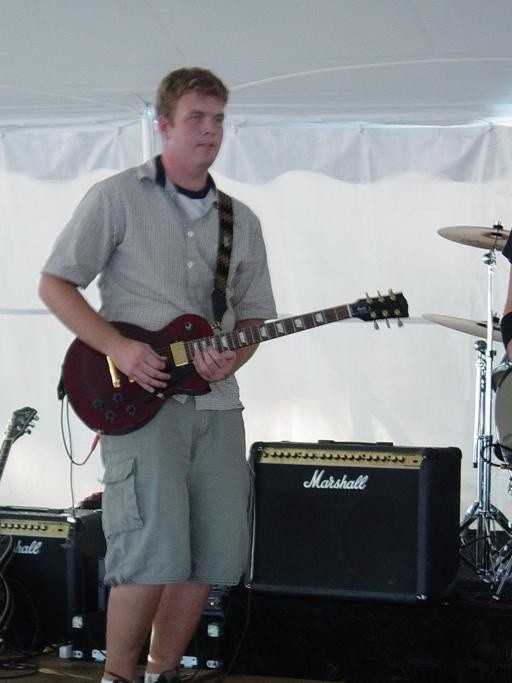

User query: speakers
[0,506,103,657]
[243,439,462,601]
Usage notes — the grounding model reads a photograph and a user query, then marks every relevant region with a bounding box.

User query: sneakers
[139,668,185,683]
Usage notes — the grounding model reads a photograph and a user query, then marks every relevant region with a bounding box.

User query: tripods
[460,254,512,604]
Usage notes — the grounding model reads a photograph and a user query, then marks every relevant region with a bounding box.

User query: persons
[37,66,277,682]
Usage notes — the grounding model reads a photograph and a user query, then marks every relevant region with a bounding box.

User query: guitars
[1,407,39,642]
[63,290,408,435]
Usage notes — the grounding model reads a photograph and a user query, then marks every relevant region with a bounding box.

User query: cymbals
[431,314,503,342]
[438,226,510,250]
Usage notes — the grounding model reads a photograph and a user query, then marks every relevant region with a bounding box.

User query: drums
[494,361,512,466]
